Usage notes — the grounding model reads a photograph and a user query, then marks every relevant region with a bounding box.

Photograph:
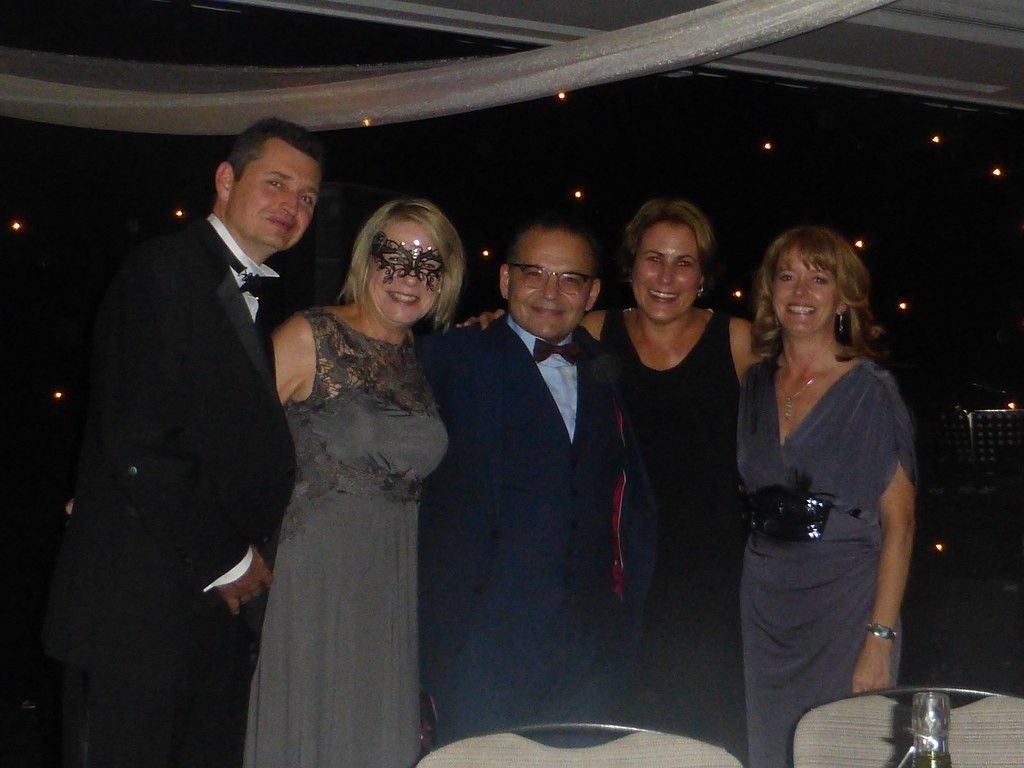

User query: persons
[41,118,917,768]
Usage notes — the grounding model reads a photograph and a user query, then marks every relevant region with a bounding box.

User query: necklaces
[779,354,815,421]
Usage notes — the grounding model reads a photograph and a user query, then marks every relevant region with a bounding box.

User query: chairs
[793,684,1023,768]
[418,722,744,768]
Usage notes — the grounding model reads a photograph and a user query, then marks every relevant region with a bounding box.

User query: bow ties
[207,220,276,299]
[533,338,580,365]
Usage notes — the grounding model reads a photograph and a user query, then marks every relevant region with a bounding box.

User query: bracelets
[864,623,896,639]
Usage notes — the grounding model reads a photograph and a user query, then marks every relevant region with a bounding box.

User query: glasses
[506,262,595,295]
